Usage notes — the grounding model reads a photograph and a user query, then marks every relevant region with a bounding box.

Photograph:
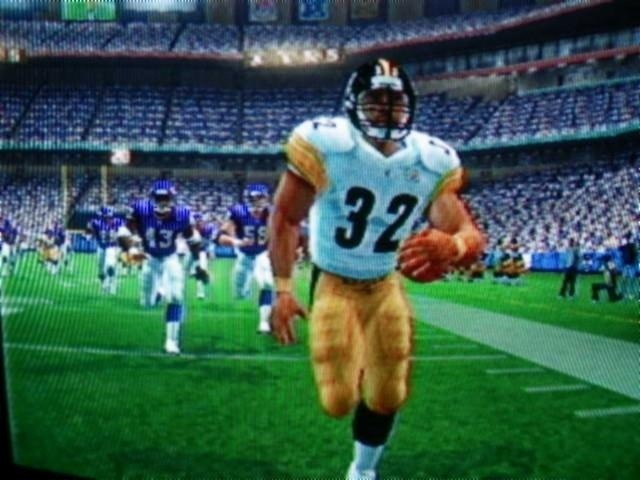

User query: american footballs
[402,229,453,282]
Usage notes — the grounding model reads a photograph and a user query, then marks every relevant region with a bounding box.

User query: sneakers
[165,337,181,354]
[257,320,270,334]
[345,461,377,480]
[196,289,206,301]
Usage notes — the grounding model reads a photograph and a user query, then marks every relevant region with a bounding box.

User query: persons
[1,0,639,144]
[558,229,639,303]
[1,181,274,353]
[453,149,640,254]
[268,59,485,479]
[442,238,532,286]
[1,172,309,233]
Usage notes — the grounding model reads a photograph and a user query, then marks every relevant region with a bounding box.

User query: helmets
[148,182,178,210]
[99,205,115,220]
[344,58,416,143]
[242,182,268,211]
